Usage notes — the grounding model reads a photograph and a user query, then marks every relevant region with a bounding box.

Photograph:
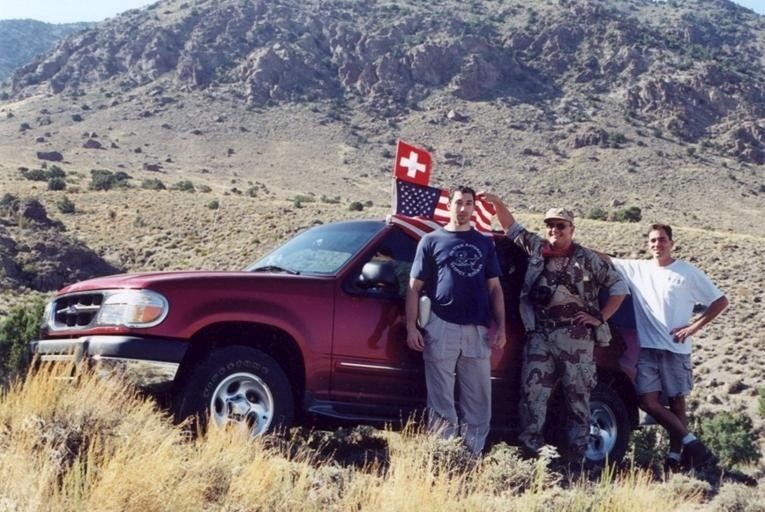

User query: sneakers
[664,440,709,471]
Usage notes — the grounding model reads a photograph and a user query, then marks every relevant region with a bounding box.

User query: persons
[598,224,729,471]
[405,187,508,461]
[476,189,630,469]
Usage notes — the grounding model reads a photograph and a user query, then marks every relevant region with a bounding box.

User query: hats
[544,207,574,225]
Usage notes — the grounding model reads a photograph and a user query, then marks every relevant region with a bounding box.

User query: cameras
[528,286,552,306]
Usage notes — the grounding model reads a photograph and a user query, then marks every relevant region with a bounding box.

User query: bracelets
[596,313,605,323]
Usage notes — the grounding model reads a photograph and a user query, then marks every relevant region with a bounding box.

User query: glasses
[546,223,570,231]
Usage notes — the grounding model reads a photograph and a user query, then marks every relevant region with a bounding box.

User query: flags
[394,140,497,263]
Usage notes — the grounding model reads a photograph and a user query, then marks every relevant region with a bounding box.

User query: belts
[537,319,577,328]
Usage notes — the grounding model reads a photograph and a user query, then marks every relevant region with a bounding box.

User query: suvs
[32,220,667,480]
